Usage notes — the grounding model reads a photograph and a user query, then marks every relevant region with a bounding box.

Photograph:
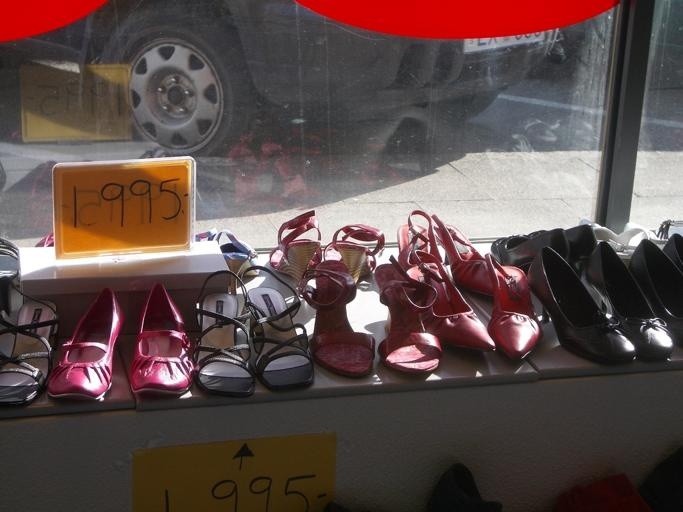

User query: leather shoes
[46,286,126,403]
[130,278,194,396]
[491,220,683,365]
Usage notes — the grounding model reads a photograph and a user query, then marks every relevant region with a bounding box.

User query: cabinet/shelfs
[0,233,683,511]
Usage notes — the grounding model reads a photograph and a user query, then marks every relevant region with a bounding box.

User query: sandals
[1,236,20,283]
[0,299,61,408]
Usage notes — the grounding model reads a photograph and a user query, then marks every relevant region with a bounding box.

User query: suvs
[0,3,563,158]
